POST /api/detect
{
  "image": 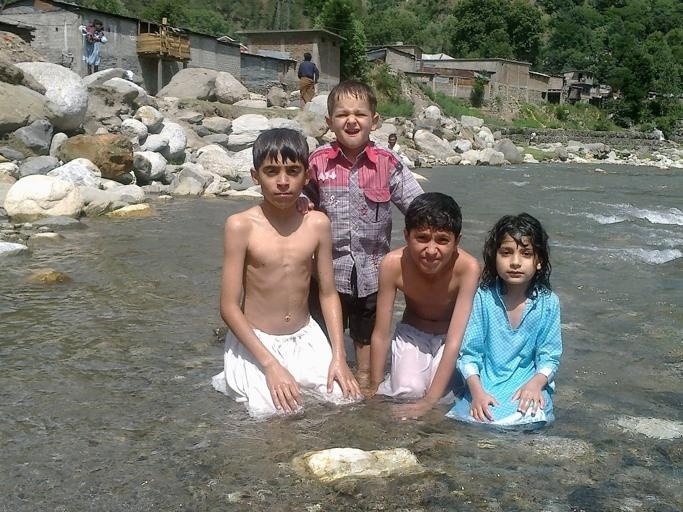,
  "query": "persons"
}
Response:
[211,126,364,423]
[369,193,481,423]
[295,79,425,393]
[387,134,404,160]
[298,52,319,110]
[448,213,564,428]
[79,18,108,75]
